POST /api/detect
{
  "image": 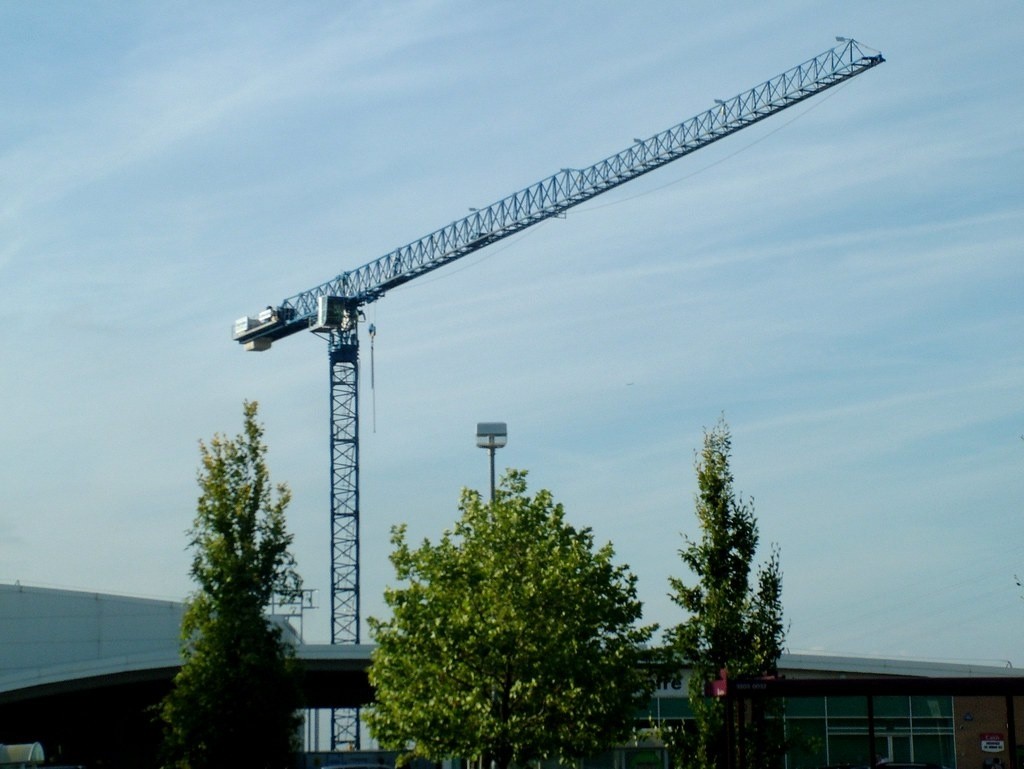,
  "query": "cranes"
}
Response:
[228,36,886,646]
